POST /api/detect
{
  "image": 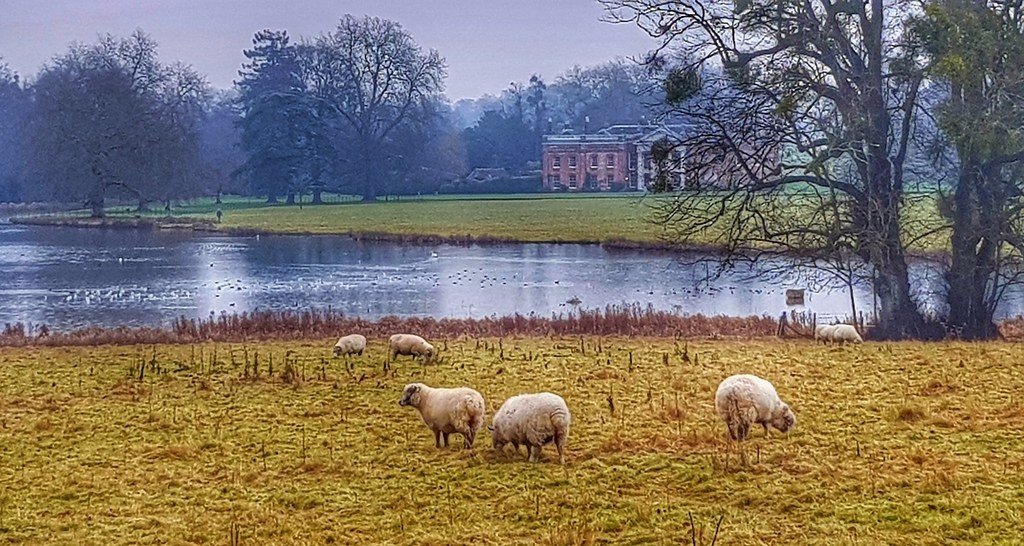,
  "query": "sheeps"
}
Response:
[386,334,434,362]
[813,323,833,346]
[715,374,797,441]
[398,382,485,449]
[333,333,366,358]
[830,324,864,345]
[486,391,571,466]
